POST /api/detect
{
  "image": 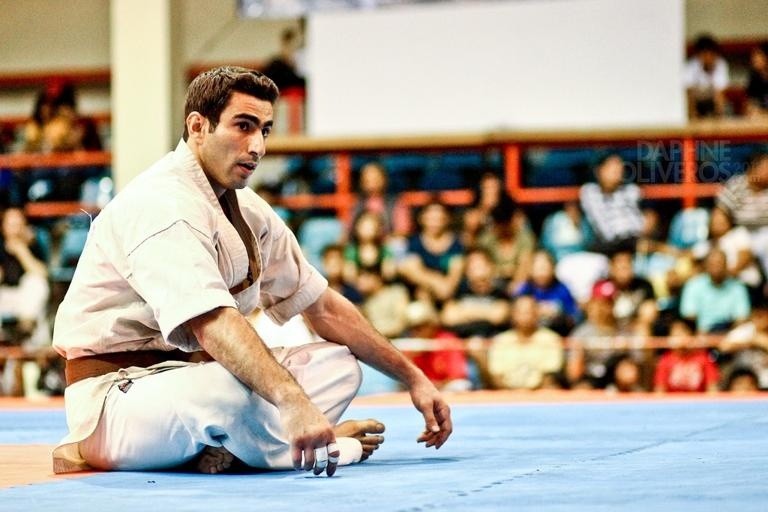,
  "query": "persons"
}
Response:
[52,63,456,475]
[319,148,768,393]
[244,28,345,285]
[687,34,768,121]
[1,77,115,395]
